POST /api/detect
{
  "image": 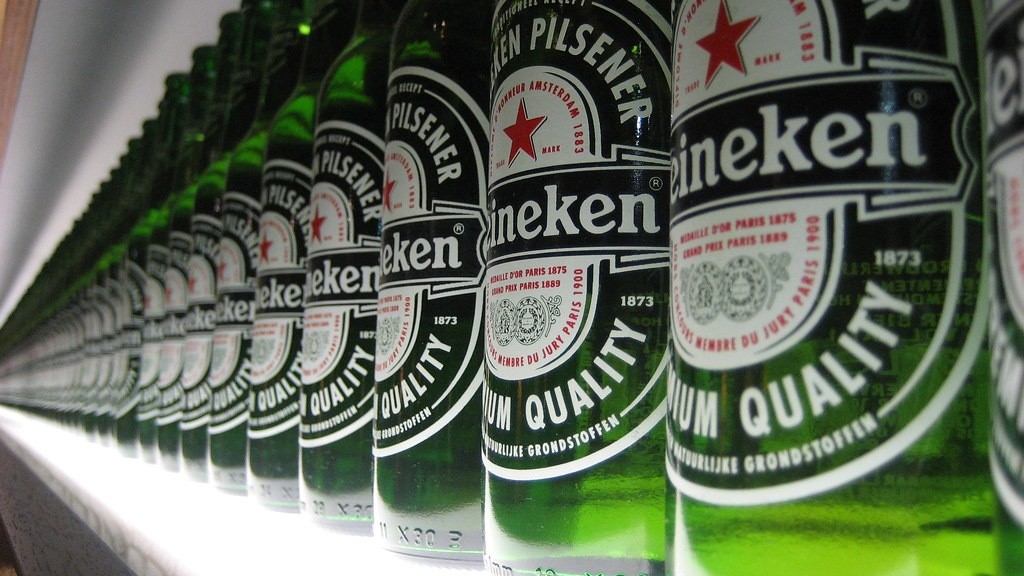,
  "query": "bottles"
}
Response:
[0,0,1024,576]
